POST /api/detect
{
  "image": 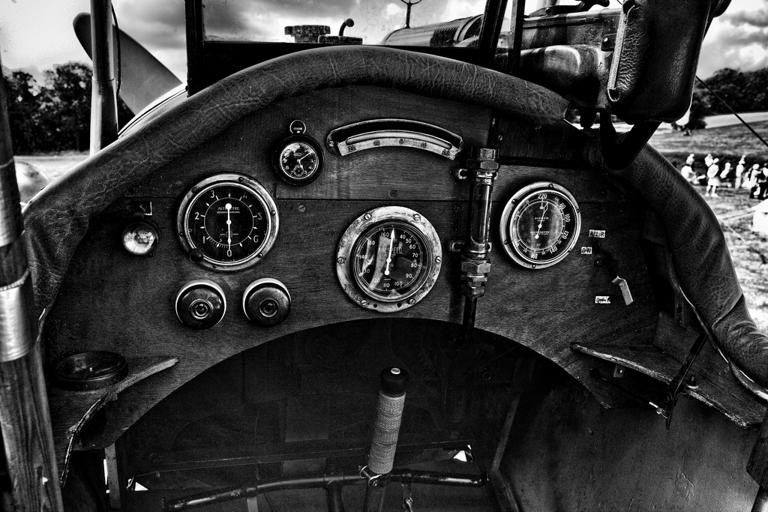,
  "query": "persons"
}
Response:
[13,162,50,212]
[671,153,768,201]
